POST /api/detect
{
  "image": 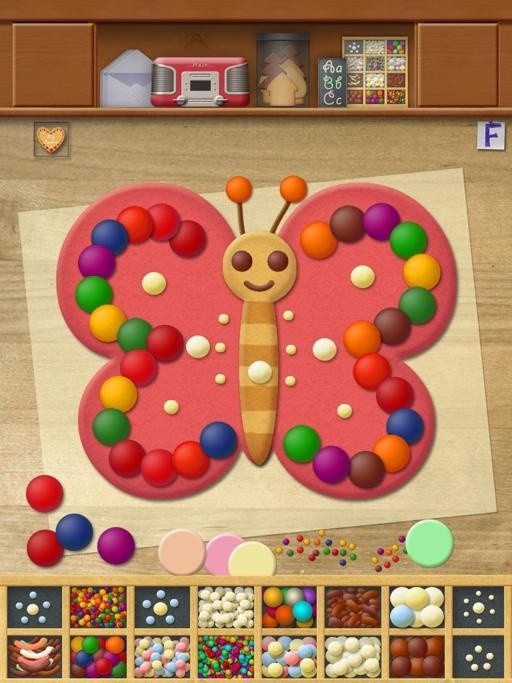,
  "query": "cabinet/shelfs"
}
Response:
[0,1,512,117]
